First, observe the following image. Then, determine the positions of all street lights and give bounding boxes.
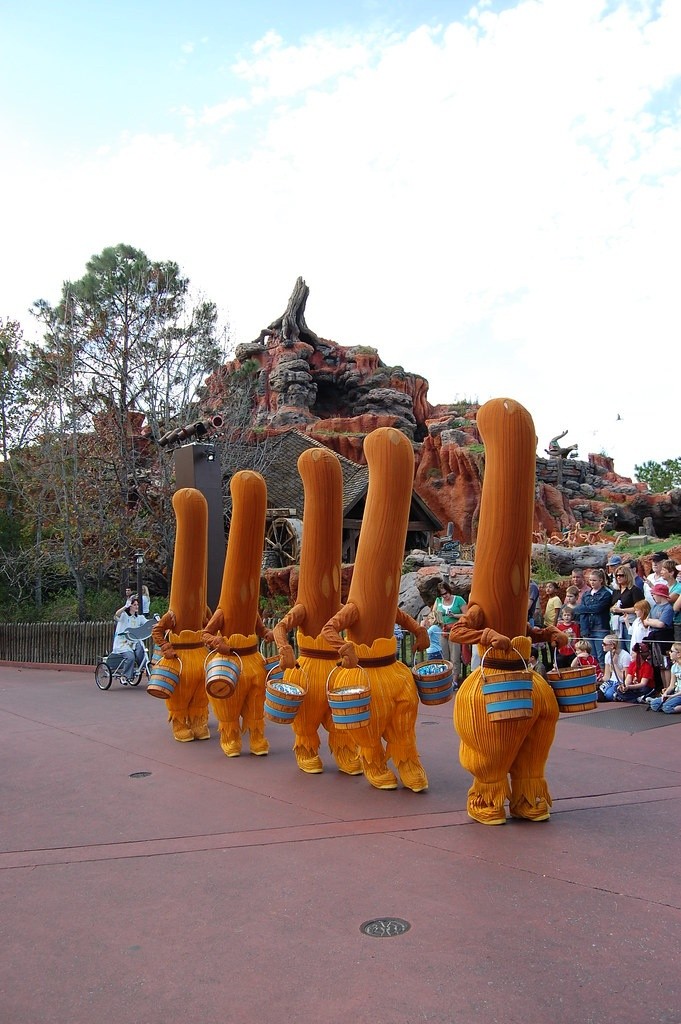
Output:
[134,548,145,615]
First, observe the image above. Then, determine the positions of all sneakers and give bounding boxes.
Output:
[119,676,129,685]
[453,681,459,691]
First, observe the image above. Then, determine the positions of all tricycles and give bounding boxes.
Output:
[95,621,156,690]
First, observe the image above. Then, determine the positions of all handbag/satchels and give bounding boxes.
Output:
[443,622,455,639]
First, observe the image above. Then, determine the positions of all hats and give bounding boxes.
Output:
[632,643,651,656]
[650,584,671,599]
[606,554,622,566]
[649,551,669,562]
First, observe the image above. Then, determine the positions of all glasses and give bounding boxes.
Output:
[439,590,448,596]
[616,573,627,577]
[602,641,612,646]
[670,649,675,653]
[125,589,131,592]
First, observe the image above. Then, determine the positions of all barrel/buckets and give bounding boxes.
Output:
[203,647,244,699]
[411,643,454,705]
[263,663,309,725]
[546,642,597,712]
[260,637,288,680]
[146,655,182,698]
[480,645,533,722]
[324,662,371,730]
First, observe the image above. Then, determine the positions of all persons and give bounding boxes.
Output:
[113,585,150,685]
[420,551,681,714]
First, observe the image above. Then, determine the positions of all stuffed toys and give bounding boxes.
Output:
[449,397,569,826]
[151,488,212,742]
[273,448,363,776]
[203,470,274,757]
[322,427,430,792]
